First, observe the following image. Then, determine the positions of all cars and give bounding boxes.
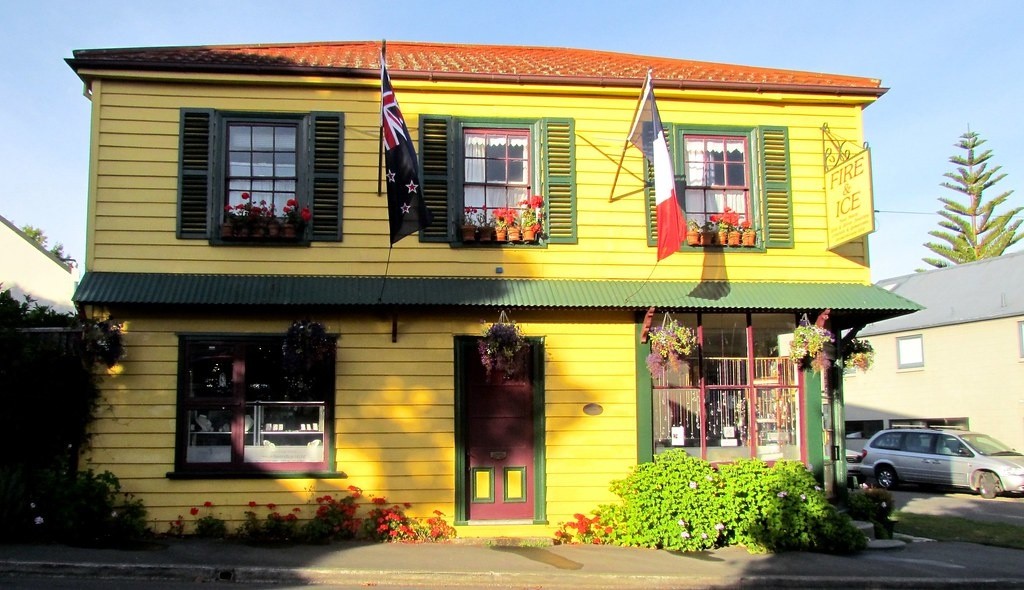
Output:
[845,436,868,486]
[861,428,1024,500]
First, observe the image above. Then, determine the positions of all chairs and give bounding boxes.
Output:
[938,440,951,455]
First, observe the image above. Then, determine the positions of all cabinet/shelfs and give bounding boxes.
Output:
[188,399,325,462]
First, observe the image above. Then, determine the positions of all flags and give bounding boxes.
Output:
[624,66,689,261]
[381,52,433,247]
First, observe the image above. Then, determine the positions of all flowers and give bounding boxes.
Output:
[843,338,874,373]
[646,319,698,379]
[225,193,314,242]
[477,319,552,385]
[688,206,752,232]
[789,326,835,377]
[452,196,547,234]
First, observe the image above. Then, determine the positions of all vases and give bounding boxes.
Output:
[495,227,506,241]
[507,228,520,241]
[700,232,714,246]
[522,227,535,241]
[462,225,477,241]
[686,231,700,246]
[478,226,493,240]
[728,231,740,246]
[716,231,726,245]
[742,231,756,246]
[221,223,296,237]
